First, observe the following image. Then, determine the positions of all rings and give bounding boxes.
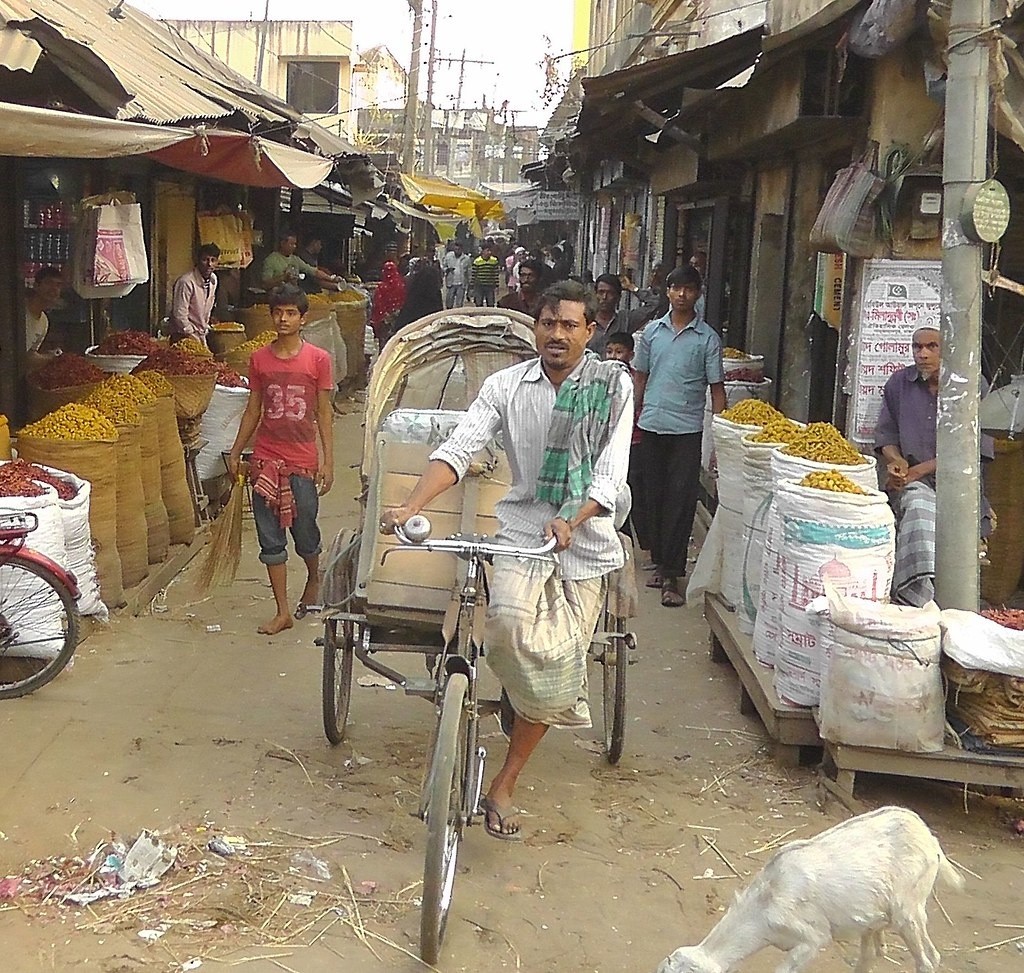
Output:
[379,521,387,529]
[315,483,327,487]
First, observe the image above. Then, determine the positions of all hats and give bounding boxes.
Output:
[514,247,525,255]
[912,315,940,342]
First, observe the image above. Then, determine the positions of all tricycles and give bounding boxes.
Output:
[316,306,639,962]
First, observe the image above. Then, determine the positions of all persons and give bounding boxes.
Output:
[873,327,996,608]
[378,278,633,838]
[228,286,334,636]
[23,268,64,349]
[262,231,726,607]
[170,243,220,347]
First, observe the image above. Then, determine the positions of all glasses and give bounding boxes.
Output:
[670,283,699,292]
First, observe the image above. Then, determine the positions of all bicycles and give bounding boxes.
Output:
[0,514,82,701]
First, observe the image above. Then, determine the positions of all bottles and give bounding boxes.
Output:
[23,263,66,289]
[21,232,71,260]
[19,198,77,229]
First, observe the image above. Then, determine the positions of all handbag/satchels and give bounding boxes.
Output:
[80,197,150,287]
[809,140,885,258]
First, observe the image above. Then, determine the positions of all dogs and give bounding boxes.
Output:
[656,804,967,973]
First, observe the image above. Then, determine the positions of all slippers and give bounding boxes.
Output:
[481,798,522,840]
[500,687,515,735]
[661,584,684,606]
[646,568,663,588]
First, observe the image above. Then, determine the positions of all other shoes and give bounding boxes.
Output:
[639,548,658,570]
